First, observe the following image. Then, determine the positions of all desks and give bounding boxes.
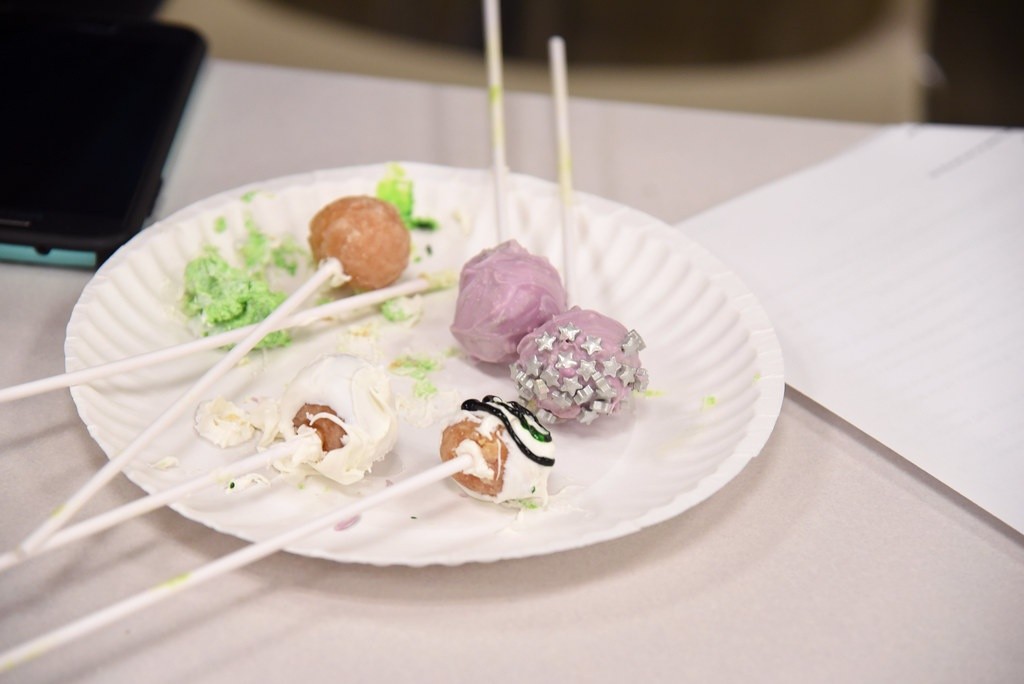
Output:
[0,63,1024,684]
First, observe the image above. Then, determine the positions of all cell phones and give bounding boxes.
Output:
[0,0,208,270]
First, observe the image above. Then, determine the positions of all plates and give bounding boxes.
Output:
[63,159,785,568]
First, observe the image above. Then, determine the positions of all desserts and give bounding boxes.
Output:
[276,193,649,503]
